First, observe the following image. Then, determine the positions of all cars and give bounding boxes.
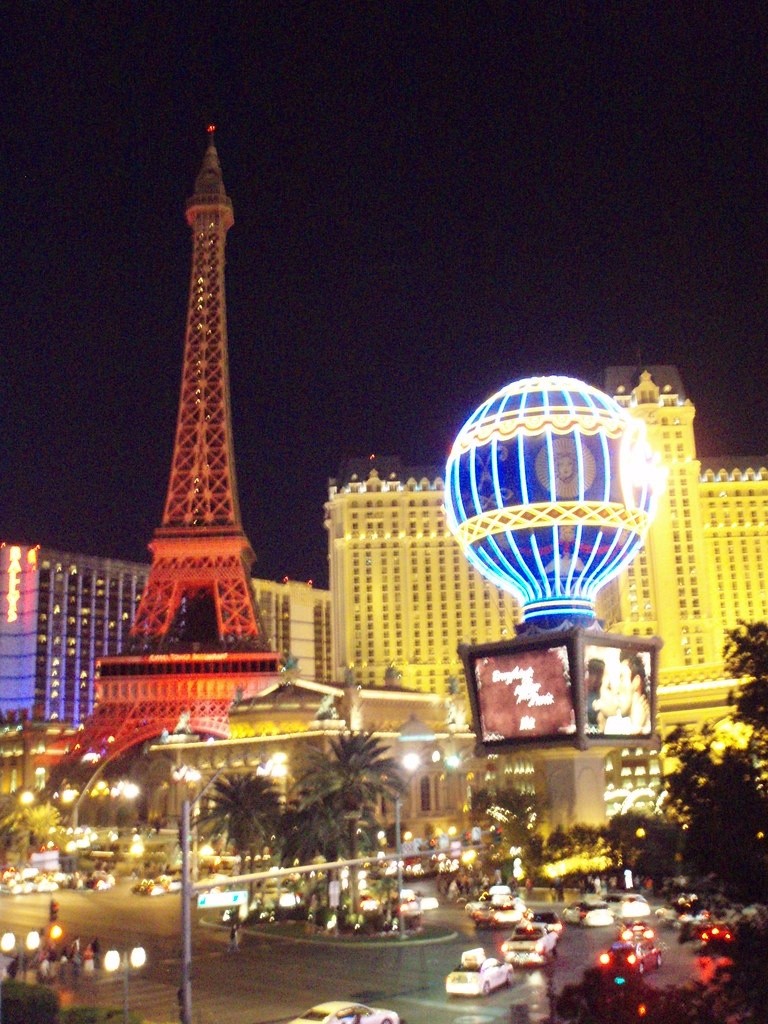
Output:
[462,884,731,969]
[287,998,402,1024]
[445,949,514,996]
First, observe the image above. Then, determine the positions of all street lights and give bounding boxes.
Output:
[179,750,288,1024]
[104,944,147,1023]
[0,929,41,979]
[394,755,461,941]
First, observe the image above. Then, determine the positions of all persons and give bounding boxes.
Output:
[435,866,673,904]
[92,857,264,877]
[585,649,650,734]
[7,937,100,978]
[226,924,239,950]
